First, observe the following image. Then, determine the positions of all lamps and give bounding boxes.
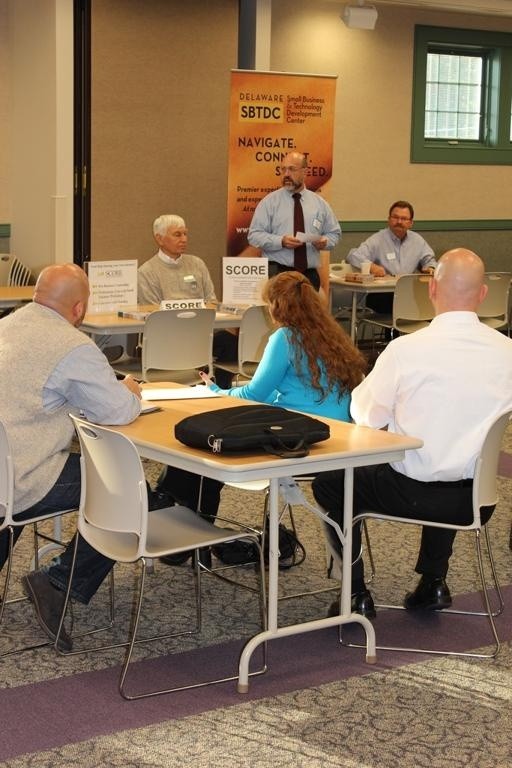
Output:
[338,0,378,30]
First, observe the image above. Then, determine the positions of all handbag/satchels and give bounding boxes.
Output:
[175,405,330,458]
[212,524,296,564]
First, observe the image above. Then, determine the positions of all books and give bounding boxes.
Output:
[140,384,223,401]
[79,400,161,419]
[345,272,396,284]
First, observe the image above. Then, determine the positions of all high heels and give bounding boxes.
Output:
[148,487,175,510]
[159,546,211,570]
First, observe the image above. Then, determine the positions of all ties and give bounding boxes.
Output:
[292,193,307,274]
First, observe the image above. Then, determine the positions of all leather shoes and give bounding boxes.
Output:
[21,566,73,651]
[328,590,376,619]
[405,576,452,612]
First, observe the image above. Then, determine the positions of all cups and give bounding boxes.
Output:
[360,262,371,275]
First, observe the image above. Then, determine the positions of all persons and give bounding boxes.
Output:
[137,214,219,306]
[0,261,142,651]
[158,271,368,571]
[247,151,342,293]
[346,200,437,314]
[311,247,511,620]
